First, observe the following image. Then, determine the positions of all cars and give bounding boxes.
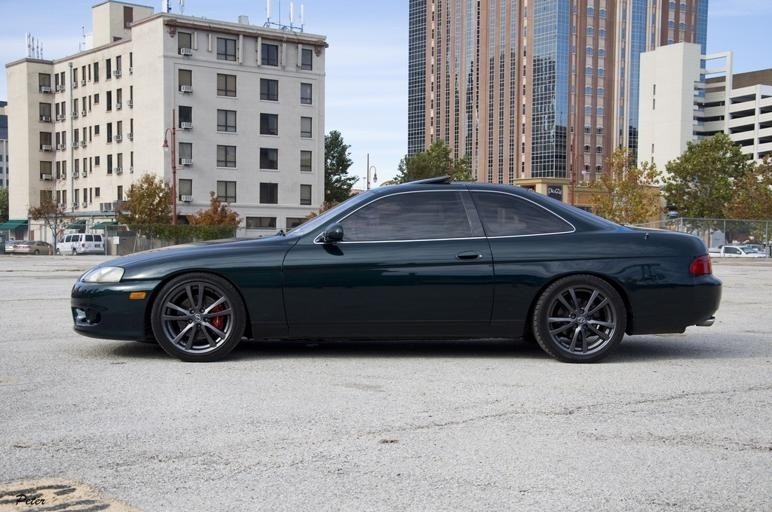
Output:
[70,175,722,363]
[3,240,56,255]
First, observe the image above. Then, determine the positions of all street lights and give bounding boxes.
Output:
[162,127,177,225]
[368,165,378,190]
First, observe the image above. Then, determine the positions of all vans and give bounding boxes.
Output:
[57,233,105,254]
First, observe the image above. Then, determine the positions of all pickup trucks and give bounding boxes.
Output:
[708,243,769,258]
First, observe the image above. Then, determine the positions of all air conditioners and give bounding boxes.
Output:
[181,48,192,56]
[181,195,192,202]
[181,86,193,92]
[181,159,192,164]
[181,121,193,128]
[41,68,133,212]
